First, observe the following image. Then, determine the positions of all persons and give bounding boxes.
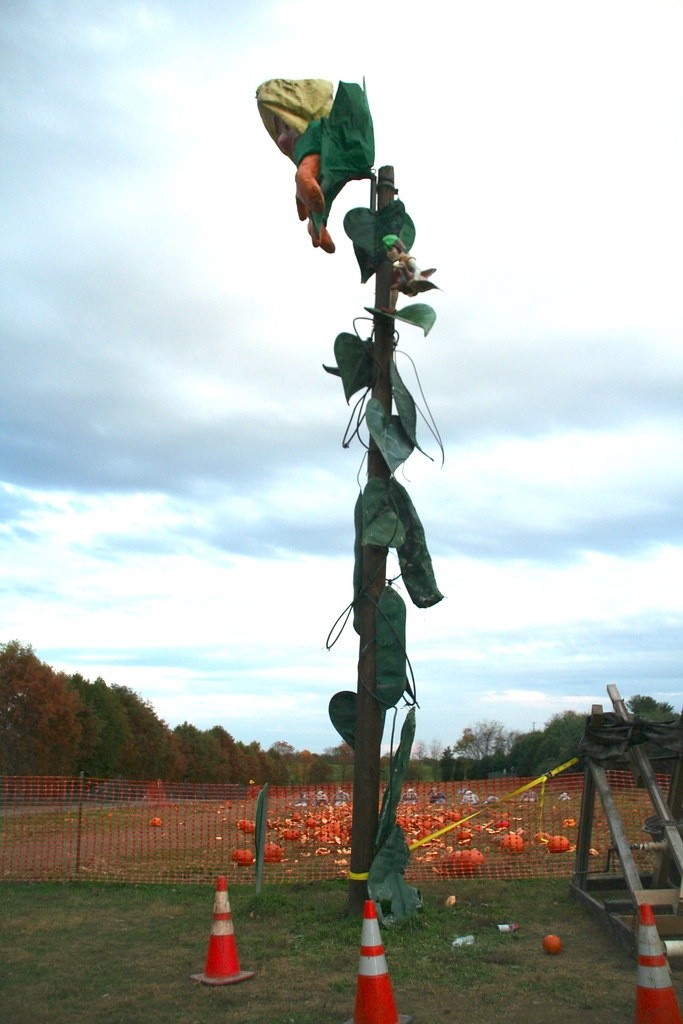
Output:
[400,788,418,805]
[333,789,349,806]
[459,789,479,806]
[312,788,328,806]
[427,785,439,796]
[558,792,571,801]
[520,789,537,802]
[295,793,309,807]
[430,791,445,805]
[483,796,499,805]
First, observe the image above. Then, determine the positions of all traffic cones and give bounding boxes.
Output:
[342,900,414,1024]
[189,875,254,987]
[634,902,683,1023]
